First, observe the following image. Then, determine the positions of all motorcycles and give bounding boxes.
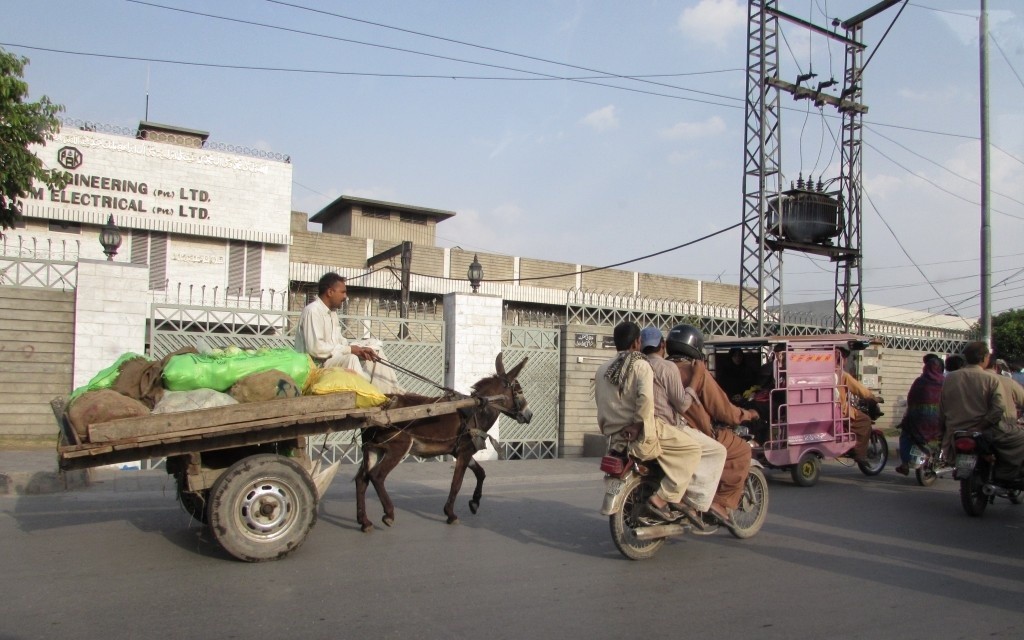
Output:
[950,429,1024,519]
[599,388,769,562]
[703,333,889,489]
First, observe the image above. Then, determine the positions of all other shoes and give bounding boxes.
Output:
[896,464,910,475]
[853,458,871,467]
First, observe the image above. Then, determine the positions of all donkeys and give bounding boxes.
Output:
[354,351,533,532]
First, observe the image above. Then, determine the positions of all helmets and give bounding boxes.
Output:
[666,324,705,360]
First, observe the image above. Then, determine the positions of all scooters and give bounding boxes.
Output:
[906,435,956,488]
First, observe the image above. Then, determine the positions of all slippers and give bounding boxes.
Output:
[646,501,678,521]
[671,502,705,531]
[708,508,738,530]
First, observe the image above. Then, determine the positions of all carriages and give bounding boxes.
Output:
[56,351,534,564]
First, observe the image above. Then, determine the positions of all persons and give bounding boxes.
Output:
[294,272,406,396]
[895,341,1024,489]
[595,322,760,531]
[721,343,786,422]
[835,347,883,463]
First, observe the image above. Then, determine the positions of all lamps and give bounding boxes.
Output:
[98,213,122,262]
[467,253,483,293]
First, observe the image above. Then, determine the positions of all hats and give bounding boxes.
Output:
[639,327,663,352]
